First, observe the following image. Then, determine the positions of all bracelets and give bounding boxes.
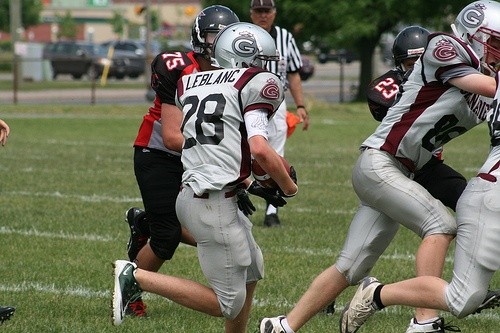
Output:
[297,106,304,108]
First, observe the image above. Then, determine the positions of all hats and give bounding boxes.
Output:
[251,0,275,10]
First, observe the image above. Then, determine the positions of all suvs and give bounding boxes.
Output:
[42,41,162,81]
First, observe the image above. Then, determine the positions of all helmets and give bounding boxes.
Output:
[450,0,500,75]
[392,26,430,66]
[210,21,280,68]
[190,5,240,55]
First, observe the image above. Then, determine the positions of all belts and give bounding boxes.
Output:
[179,185,237,199]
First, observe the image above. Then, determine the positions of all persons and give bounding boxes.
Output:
[111,22,299,333]
[339,70,500,333]
[258,0,500,333]
[247,0,310,227]
[0,119,15,324]
[366,25,500,314]
[124,4,240,316]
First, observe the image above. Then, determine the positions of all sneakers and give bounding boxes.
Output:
[339,276,383,333]
[112,259,146,326]
[406,316,461,333]
[125,206,148,261]
[257,315,290,333]
[264,209,280,226]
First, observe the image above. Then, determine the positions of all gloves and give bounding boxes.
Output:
[247,179,288,208]
[238,188,257,216]
[277,166,300,198]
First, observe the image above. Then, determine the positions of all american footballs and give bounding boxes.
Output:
[251,154,291,189]
